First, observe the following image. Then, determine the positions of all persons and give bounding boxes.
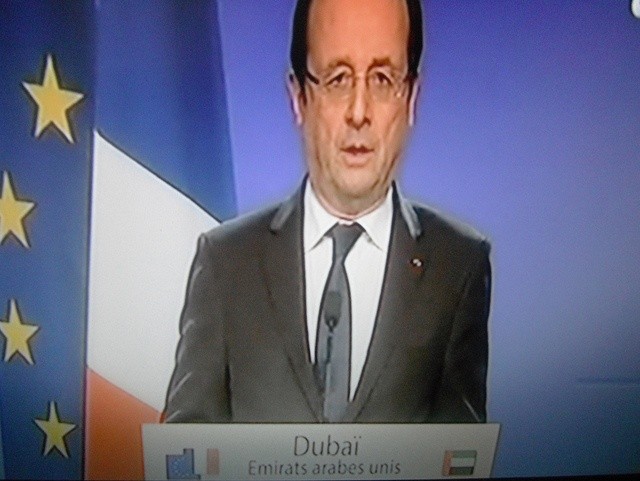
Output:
[159,0,493,424]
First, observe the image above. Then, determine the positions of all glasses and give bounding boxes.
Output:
[299,64,410,103]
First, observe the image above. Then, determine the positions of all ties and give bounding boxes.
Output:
[313,222,366,421]
